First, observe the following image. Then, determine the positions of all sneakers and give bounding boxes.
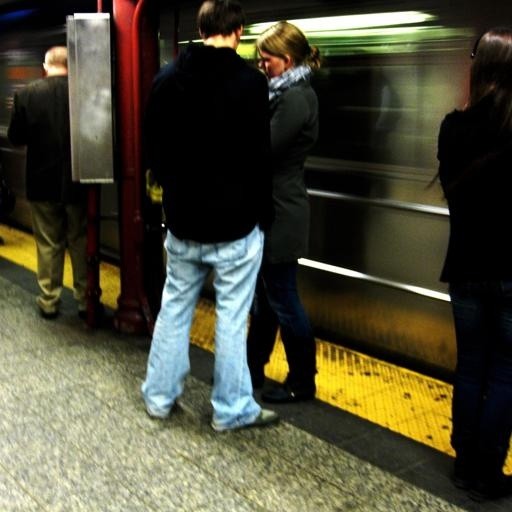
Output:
[39,305,59,320]
[261,380,294,404]
[140,381,169,420]
[211,409,278,432]
[78,302,104,322]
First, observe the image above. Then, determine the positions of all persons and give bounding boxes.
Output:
[251,21,321,405]
[6,45,109,322]
[137,0,284,432]
[424,25,512,505]
